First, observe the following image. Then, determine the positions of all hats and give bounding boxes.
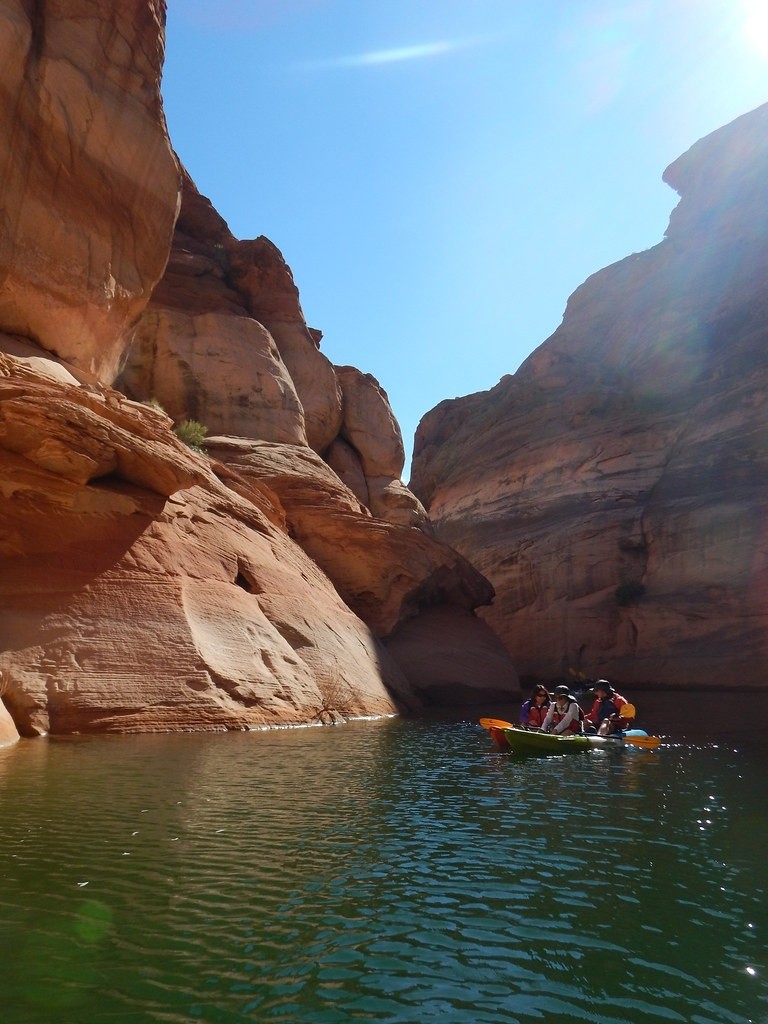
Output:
[548,685,577,702]
[588,680,615,692]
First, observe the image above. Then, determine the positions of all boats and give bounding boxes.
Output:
[502,727,661,757]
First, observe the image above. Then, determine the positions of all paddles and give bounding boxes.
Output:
[615,703,636,719]
[478,715,661,748]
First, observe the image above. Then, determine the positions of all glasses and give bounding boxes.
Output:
[555,696,565,700]
[537,694,547,697]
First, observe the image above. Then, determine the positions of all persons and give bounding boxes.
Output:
[520,684,558,728]
[583,679,630,735]
[538,685,584,736]
